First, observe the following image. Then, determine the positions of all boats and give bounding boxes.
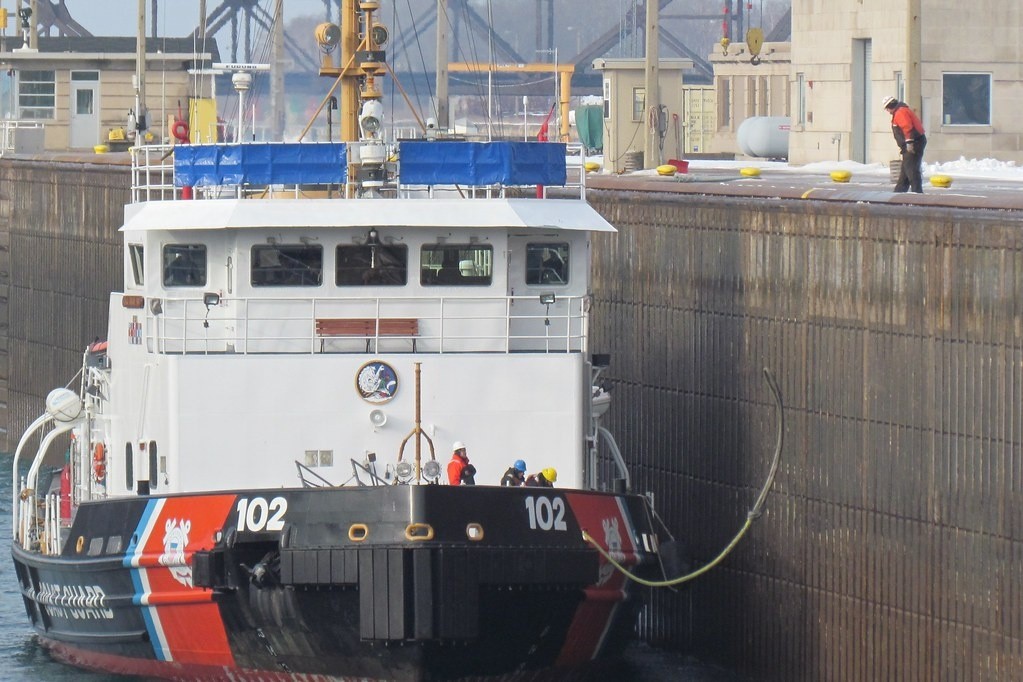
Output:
[7,1,679,682]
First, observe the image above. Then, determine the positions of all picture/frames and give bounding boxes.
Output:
[354,359,400,405]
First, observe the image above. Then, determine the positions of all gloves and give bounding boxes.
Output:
[905,142,916,154]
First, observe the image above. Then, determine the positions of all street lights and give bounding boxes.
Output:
[503,30,519,54]
[535,47,561,142]
[522,95,530,142]
[567,24,581,55]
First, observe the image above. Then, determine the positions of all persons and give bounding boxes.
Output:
[525,468,557,487]
[883,96,927,193]
[529,246,566,285]
[447,442,475,485]
[438,257,462,279]
[500,460,526,486]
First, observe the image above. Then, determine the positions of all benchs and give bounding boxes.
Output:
[314,319,420,353]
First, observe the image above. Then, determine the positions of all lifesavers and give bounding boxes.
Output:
[172,121,189,138]
[95,444,104,475]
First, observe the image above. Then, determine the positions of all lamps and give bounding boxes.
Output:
[539,290,556,352]
[392,462,417,484]
[202,293,220,352]
[420,460,442,484]
[357,100,385,165]
[314,22,341,67]
[370,22,391,50]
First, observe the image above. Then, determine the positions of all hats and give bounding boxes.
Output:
[881,96,895,110]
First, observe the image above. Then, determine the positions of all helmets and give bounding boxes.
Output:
[514,460,527,471]
[541,467,557,482]
[453,442,466,450]
